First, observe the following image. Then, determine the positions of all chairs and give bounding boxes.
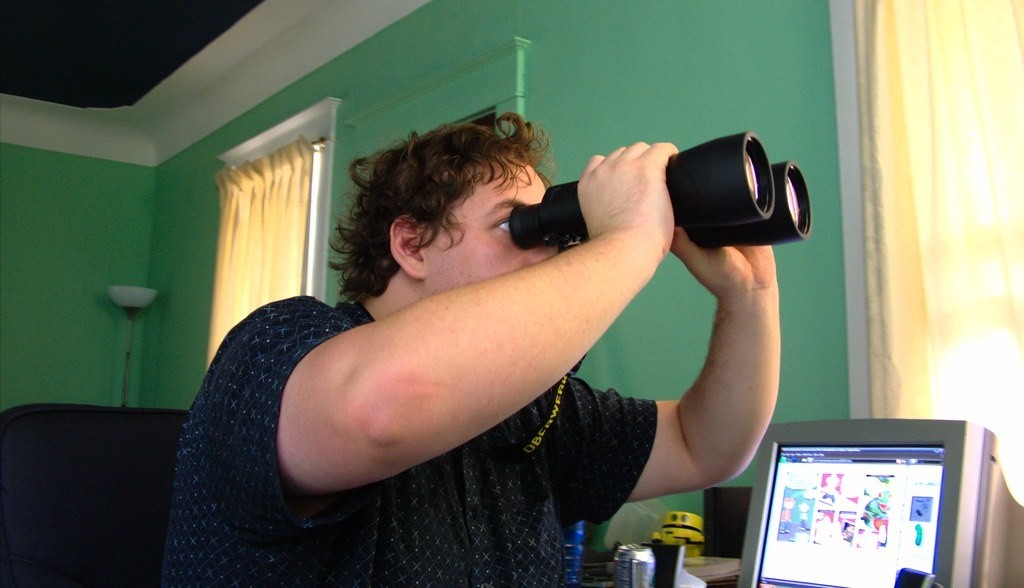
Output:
[0,402,191,588]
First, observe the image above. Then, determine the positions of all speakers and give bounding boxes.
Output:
[703,486,752,559]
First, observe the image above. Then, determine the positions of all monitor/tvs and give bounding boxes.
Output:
[735,419,1024,588]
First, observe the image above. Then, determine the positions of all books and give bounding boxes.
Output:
[683,557,741,582]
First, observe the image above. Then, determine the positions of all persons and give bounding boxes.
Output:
[160,111,782,588]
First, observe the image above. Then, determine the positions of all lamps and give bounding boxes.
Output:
[107,285,160,407]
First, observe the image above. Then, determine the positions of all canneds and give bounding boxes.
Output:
[613,543,657,588]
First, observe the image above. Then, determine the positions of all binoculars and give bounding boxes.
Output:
[508,129,814,249]
